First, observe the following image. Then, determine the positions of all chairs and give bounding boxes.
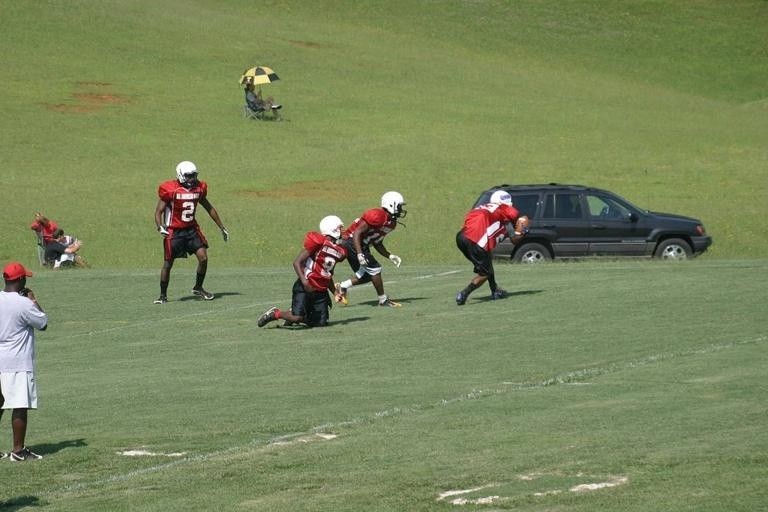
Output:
[242,87,283,121]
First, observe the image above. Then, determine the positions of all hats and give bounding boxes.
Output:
[2,261,33,280]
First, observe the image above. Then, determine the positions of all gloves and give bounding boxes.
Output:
[356,252,370,267]
[222,228,229,242]
[156,224,172,238]
[521,223,530,236]
[387,254,402,267]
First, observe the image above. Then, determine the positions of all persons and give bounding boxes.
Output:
[454,188,530,307]
[246,83,280,120]
[30,212,91,270]
[334,189,407,308]
[257,214,350,328]
[0,260,50,462]
[153,158,229,306]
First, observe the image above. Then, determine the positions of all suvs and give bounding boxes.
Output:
[471,180,713,264]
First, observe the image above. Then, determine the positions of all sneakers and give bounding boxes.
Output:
[192,287,215,300]
[0,445,43,462]
[333,281,348,306]
[456,289,468,306]
[153,295,168,304]
[256,306,280,327]
[377,294,402,308]
[490,288,510,300]
[271,104,282,109]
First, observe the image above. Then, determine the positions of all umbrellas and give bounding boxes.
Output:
[238,64,281,119]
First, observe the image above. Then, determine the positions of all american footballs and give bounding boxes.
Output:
[515,216,528,236]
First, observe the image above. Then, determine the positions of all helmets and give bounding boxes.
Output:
[176,160,199,187]
[489,190,513,207]
[319,215,344,240]
[381,191,405,215]
